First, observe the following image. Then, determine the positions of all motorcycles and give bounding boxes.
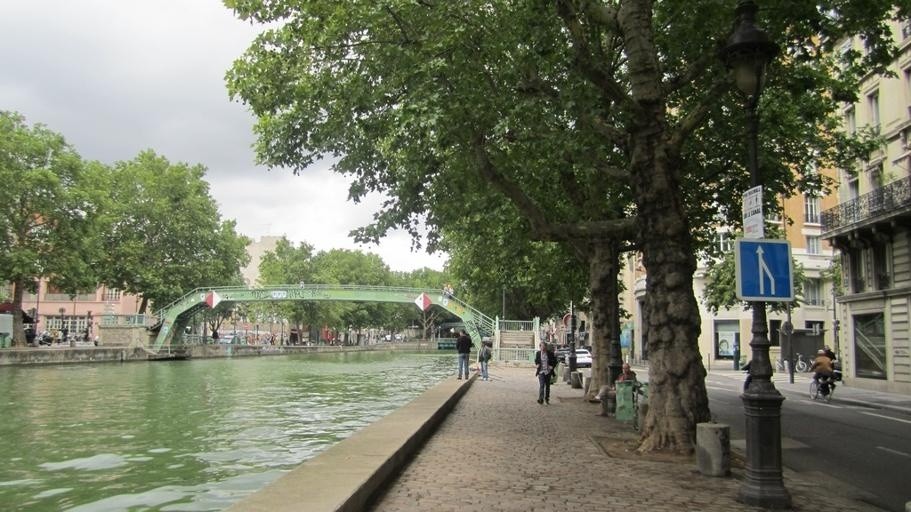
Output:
[776,352,816,373]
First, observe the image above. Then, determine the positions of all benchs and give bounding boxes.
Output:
[602,372,641,414]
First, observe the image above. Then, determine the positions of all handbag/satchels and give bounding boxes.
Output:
[479,354,483,362]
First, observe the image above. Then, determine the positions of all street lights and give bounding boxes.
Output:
[244,325,249,344]
[717,1,798,512]
[438,326,442,342]
[257,322,260,350]
[349,326,353,345]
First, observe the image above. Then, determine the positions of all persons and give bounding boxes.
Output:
[448,285,454,298]
[742,359,752,391]
[443,284,448,296]
[456,329,471,379]
[535,342,556,404]
[825,345,836,370]
[809,349,832,392]
[590,363,636,416]
[478,342,492,380]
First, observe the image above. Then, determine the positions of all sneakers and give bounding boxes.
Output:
[588,398,608,417]
[457,377,469,380]
[536,398,551,406]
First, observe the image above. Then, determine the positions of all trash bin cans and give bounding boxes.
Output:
[32,338,39,347]
[770,346,782,371]
[614,380,638,421]
[0,333,12,348]
[240,336,246,344]
[70,340,76,347]
[357,334,365,345]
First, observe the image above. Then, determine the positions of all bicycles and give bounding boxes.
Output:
[808,360,836,402]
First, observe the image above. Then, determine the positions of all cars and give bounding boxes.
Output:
[24,328,94,346]
[219,334,241,344]
[381,332,404,342]
[302,331,310,343]
[549,343,593,366]
